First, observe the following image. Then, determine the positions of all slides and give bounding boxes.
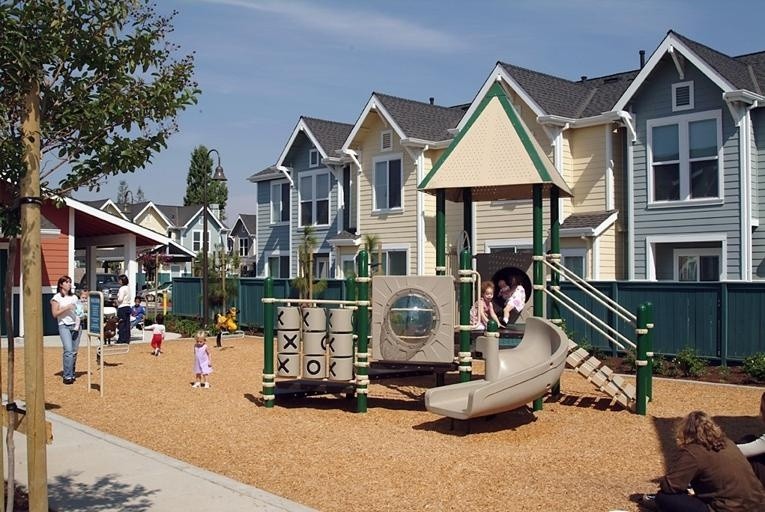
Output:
[425,317,568,420]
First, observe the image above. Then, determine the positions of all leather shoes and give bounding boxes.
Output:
[63,377,72,384]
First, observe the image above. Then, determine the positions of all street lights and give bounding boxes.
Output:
[120,189,134,224]
[203,146,228,332]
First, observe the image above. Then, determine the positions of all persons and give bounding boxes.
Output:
[734,392,765,487]
[192,331,213,388]
[469,281,507,328]
[50,276,82,384]
[112,275,131,344]
[130,296,146,330]
[71,289,88,332]
[499,275,526,326]
[144,314,166,356]
[656,411,765,512]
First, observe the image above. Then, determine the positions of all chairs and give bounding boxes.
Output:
[129,298,148,341]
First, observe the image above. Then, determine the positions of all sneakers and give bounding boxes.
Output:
[204,382,209,389]
[192,382,200,388]
[155,348,160,356]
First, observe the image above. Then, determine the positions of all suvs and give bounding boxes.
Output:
[81,273,123,306]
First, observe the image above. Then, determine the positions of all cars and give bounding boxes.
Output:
[135,281,172,303]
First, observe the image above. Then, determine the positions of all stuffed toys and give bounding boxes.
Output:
[496,280,511,300]
